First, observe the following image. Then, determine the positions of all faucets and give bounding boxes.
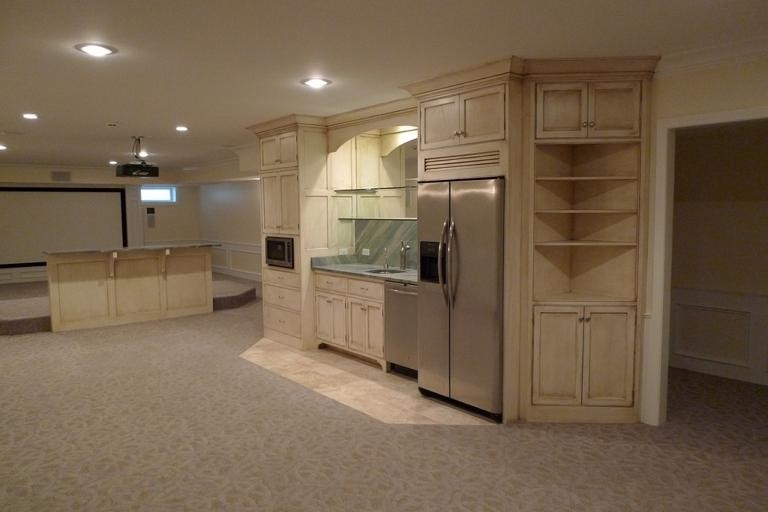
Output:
[400,241,407,270]
[405,244,411,249]
[384,247,388,267]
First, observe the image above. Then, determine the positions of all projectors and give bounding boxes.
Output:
[115,164,159,177]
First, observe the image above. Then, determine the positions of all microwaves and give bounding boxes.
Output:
[265,236,294,269]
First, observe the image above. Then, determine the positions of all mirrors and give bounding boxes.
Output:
[356,188,418,219]
[379,128,418,187]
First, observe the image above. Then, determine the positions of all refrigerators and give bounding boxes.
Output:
[417,177,506,418]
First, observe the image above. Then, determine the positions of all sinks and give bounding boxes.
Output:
[365,268,404,274]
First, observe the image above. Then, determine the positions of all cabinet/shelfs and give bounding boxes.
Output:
[535,80,642,138]
[534,141,638,303]
[260,171,328,235]
[315,272,347,347]
[420,83,506,150]
[263,267,313,339]
[328,126,381,191]
[349,278,384,360]
[532,305,636,407]
[259,128,328,170]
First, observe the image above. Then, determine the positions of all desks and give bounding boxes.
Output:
[41,240,222,333]
